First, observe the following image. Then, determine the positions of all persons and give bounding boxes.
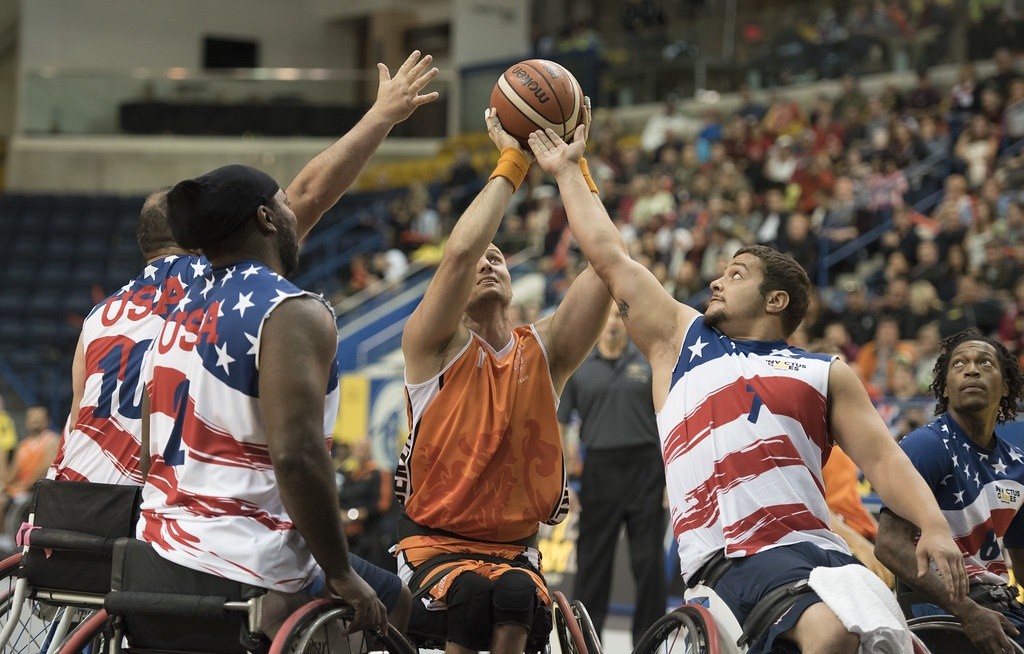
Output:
[530,125,968,654]
[0,0,1024,591]
[387,109,631,654]
[874,327,1024,654]
[134,163,412,637]
[46,48,439,486]
[555,301,665,653]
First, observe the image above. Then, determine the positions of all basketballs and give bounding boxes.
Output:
[488,59,585,156]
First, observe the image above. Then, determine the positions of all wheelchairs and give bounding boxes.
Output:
[630,568,1024,654]
[0,476,604,654]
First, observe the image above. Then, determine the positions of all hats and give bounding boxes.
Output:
[166,164,279,250]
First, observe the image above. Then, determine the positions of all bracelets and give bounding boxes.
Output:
[488,147,530,191]
[580,158,599,194]
